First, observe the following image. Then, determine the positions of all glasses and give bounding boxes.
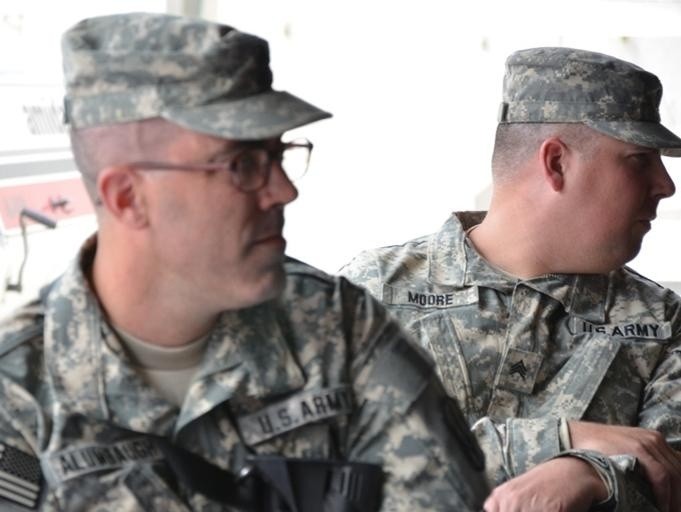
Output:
[126,135,313,194]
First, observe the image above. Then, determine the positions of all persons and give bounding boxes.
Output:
[1,12,487,511]
[331,46,681,509]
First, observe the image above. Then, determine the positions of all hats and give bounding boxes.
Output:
[59,10,333,144]
[498,46,681,158]
